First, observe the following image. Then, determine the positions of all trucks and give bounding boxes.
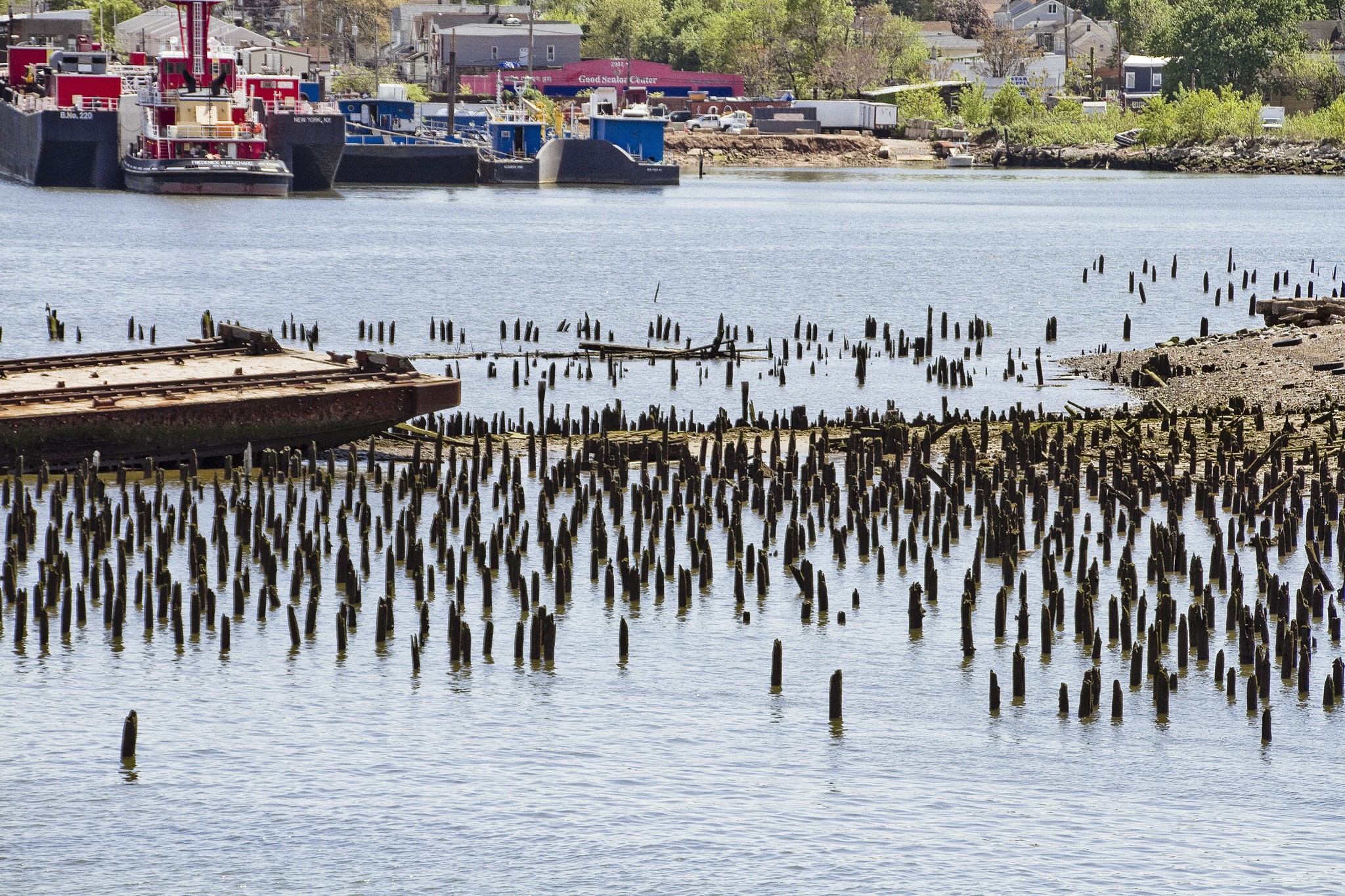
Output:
[1259,107,1285,131]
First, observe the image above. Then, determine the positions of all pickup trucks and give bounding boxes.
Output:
[684,114,748,132]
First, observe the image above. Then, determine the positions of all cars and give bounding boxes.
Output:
[727,123,746,133]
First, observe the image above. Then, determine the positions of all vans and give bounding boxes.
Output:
[664,110,693,122]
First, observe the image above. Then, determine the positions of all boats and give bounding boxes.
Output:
[1113,127,1151,148]
[120,0,295,198]
